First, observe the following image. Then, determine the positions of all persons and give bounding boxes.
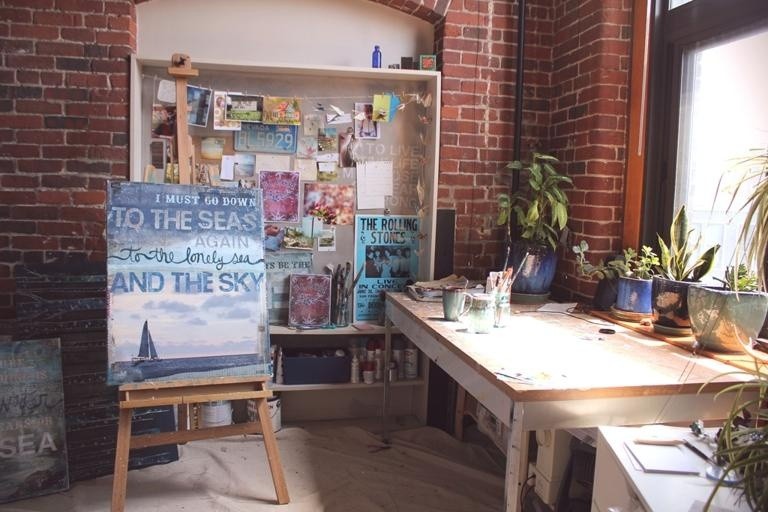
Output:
[360,105,378,138]
[367,246,410,277]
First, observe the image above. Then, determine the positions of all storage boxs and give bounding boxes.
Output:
[280,343,353,385]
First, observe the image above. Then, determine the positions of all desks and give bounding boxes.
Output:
[378,284,768,512]
[589,423,768,511]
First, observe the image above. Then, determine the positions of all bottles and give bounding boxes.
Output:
[371,44,383,68]
[347,336,418,385]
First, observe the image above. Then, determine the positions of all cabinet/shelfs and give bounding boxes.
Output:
[128,54,444,441]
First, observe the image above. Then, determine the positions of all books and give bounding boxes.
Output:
[623,438,701,474]
[405,274,488,303]
[287,273,333,328]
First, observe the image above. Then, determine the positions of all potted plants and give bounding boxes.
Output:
[686,263,768,354]
[573,237,621,310]
[495,154,571,293]
[610,244,658,313]
[649,205,720,336]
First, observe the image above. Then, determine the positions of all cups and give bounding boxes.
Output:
[472,292,494,334]
[486,292,511,328]
[442,286,474,322]
